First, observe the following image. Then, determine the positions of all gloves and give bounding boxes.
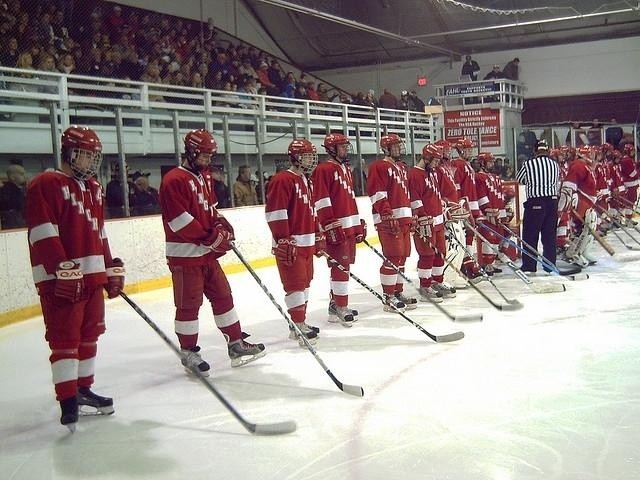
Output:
[314,233,327,258]
[104,258,124,299]
[382,216,400,235]
[276,239,297,267]
[410,215,433,239]
[444,201,513,230]
[201,218,235,254]
[355,220,367,244]
[53,261,84,303]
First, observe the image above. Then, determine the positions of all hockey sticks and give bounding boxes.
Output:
[467,220,589,293]
[230,242,365,395]
[321,220,522,343]
[118,290,296,434]
[571,193,640,262]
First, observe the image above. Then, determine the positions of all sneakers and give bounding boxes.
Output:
[460,263,502,280]
[557,216,638,252]
[288,321,320,339]
[520,265,536,272]
[419,282,456,299]
[60,386,112,424]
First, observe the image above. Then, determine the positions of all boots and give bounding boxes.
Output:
[328,290,359,321]
[180,346,210,370]
[383,289,417,307]
[224,333,265,358]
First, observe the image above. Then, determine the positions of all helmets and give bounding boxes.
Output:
[288,141,318,174]
[477,152,496,162]
[324,133,353,162]
[185,129,217,171]
[381,135,406,163]
[60,127,103,182]
[423,138,473,172]
[536,140,634,166]
